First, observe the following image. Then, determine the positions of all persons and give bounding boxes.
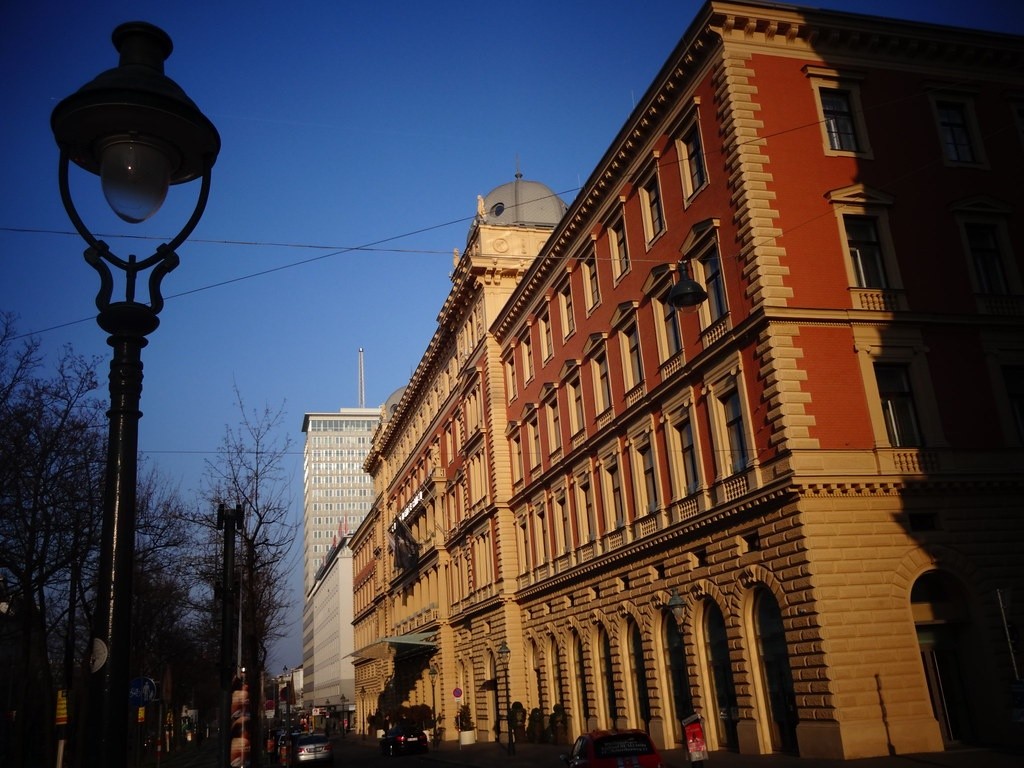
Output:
[181,719,189,743]
[143,682,151,701]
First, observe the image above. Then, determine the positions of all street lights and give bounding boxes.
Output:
[428,665,439,751]
[46,16,224,768]
[498,640,517,755]
[358,686,367,740]
[340,694,346,738]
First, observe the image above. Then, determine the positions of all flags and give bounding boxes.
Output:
[394,515,420,569]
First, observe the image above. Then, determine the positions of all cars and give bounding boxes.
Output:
[266,718,336,768]
[560,728,668,768]
[379,724,429,757]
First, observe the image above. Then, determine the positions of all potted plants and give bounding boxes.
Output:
[455,702,476,744]
